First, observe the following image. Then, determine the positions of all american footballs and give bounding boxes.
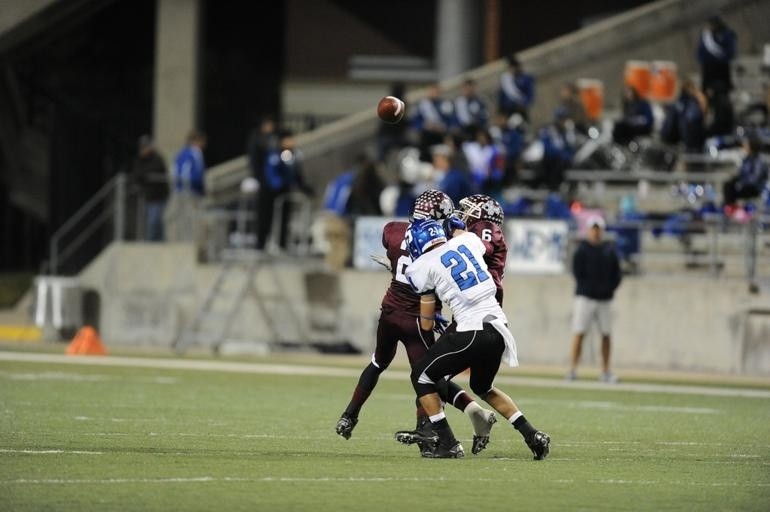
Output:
[379,96,405,124]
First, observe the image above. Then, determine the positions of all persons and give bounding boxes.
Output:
[333,188,454,442]
[402,216,550,461]
[253,128,318,250]
[246,115,277,179]
[563,214,624,384]
[136,134,170,239]
[168,130,209,246]
[320,13,770,280]
[395,192,507,454]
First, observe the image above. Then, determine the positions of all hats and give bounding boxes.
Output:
[587,215,607,230]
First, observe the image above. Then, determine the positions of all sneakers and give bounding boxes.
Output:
[600,372,619,382]
[532,431,550,459]
[335,412,358,439]
[472,410,496,455]
[394,422,438,445]
[567,369,576,380]
[422,441,464,458]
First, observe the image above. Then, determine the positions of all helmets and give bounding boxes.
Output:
[405,218,447,261]
[413,189,455,221]
[459,194,504,223]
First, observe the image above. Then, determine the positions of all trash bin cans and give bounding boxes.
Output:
[35,276,101,341]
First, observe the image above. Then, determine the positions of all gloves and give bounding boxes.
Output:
[370,251,391,270]
[443,217,465,240]
[432,314,450,334]
